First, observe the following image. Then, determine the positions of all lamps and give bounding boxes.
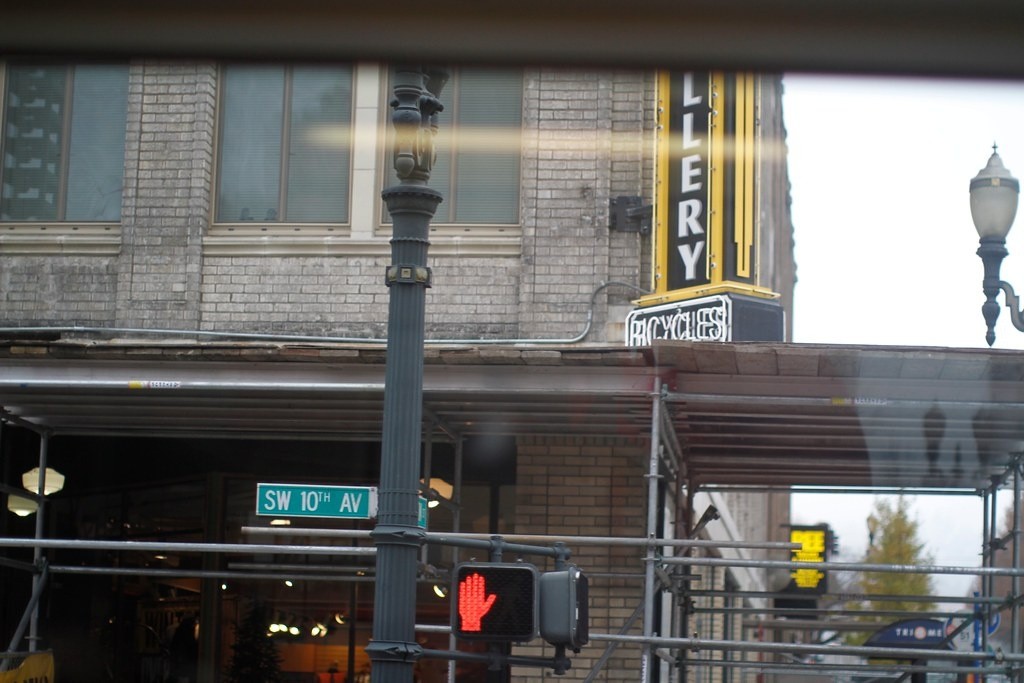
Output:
[432,583,449,599]
[968,141,1024,346]
[419,478,453,508]
[303,623,320,637]
[289,616,304,636]
[278,613,294,633]
[269,610,284,633]
[22,467,65,496]
[7,495,38,517]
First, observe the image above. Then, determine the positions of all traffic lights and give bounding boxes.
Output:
[452,563,539,644]
[538,568,590,653]
